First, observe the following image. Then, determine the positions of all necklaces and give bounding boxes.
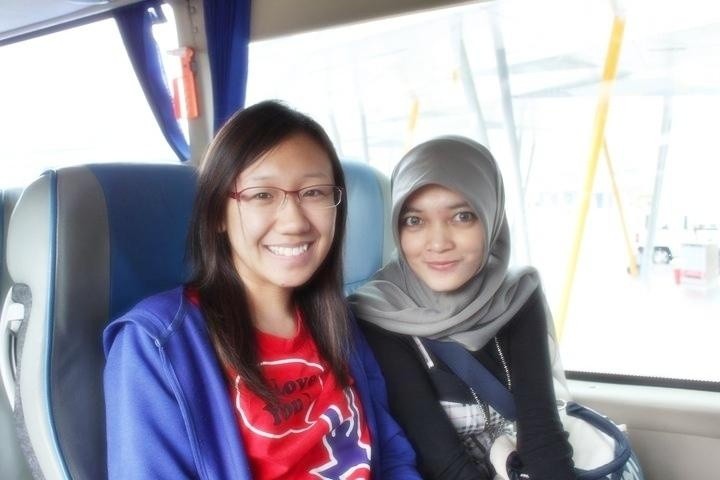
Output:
[470,335,511,441]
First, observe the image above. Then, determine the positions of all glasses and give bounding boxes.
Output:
[229,183,345,215]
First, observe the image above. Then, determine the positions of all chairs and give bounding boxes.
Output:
[2,159,220,480]
[332,153,402,305]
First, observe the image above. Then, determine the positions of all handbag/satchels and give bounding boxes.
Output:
[491,401,647,480]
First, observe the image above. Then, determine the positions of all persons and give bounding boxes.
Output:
[101,99,423,480]
[346,136,643,480]
